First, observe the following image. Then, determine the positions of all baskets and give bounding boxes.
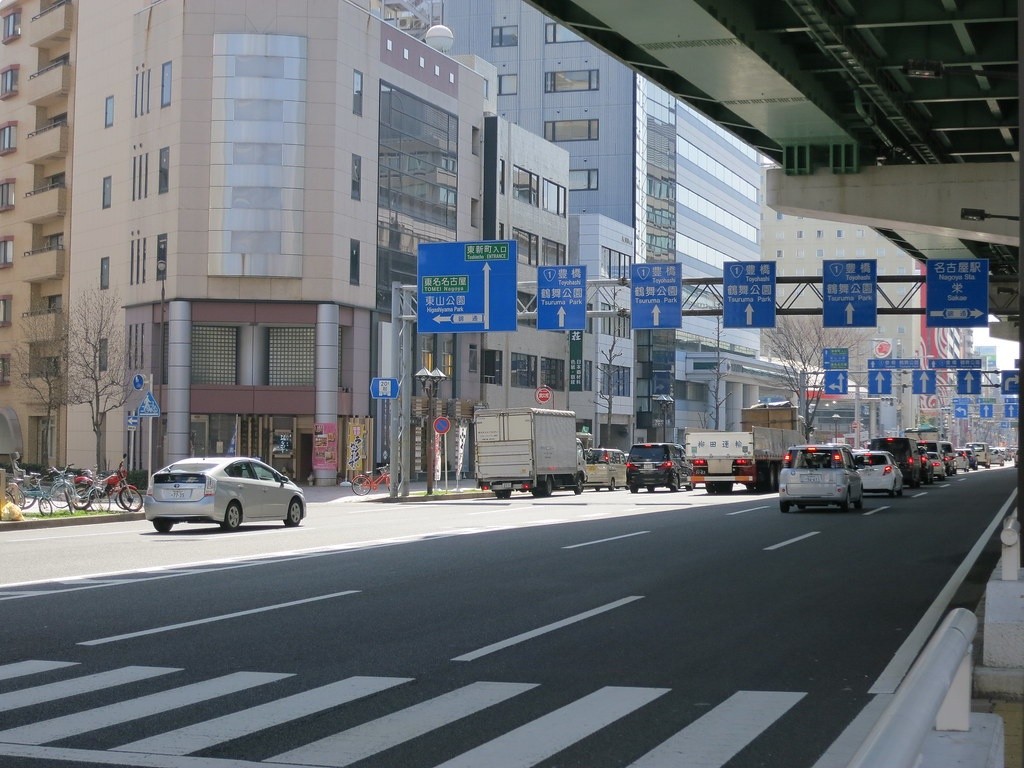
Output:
[95,474,108,487]
[53,472,74,486]
[380,467,390,475]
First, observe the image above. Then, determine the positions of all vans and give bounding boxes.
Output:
[918,440,957,475]
[966,443,991,468]
[869,438,924,489]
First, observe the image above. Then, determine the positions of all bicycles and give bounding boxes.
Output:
[351,464,392,496]
[7,462,132,513]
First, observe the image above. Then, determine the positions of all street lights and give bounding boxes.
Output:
[657,395,675,443]
[412,366,447,495]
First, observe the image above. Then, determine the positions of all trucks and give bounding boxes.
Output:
[686,406,814,495]
[473,406,587,500]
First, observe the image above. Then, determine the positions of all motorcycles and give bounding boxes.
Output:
[72,452,143,512]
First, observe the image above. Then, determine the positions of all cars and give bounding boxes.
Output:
[584,437,1019,513]
[145,454,307,532]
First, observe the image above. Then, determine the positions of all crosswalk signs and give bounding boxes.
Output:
[139,390,160,417]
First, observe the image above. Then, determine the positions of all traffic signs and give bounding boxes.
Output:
[723,261,776,329]
[868,359,983,369]
[631,262,682,330]
[823,348,849,369]
[537,266,586,331]
[417,239,518,333]
[823,259,877,328]
[926,259,988,327]
